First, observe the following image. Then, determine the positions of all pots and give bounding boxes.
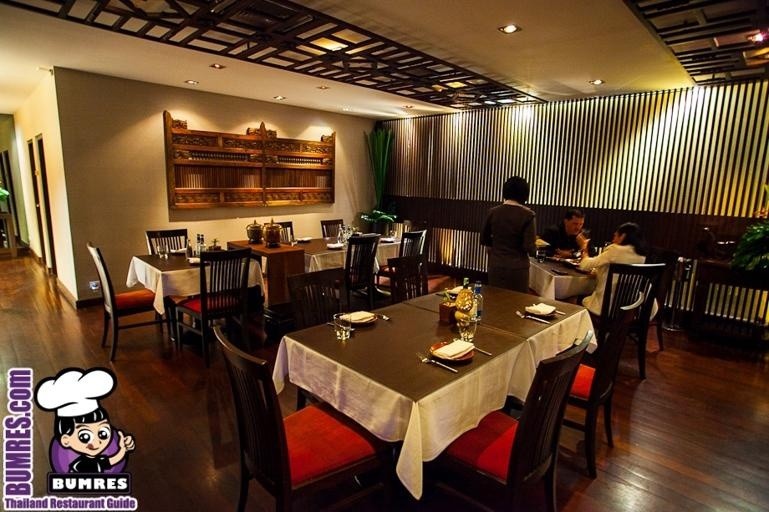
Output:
[246,219,263,239]
[262,219,285,243]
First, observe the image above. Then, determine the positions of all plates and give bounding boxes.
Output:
[170,251,186,256]
[188,258,209,265]
[328,245,344,249]
[430,342,474,362]
[296,237,312,242]
[340,314,377,324]
[524,306,558,317]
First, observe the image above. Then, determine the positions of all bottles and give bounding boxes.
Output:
[200,235,206,252]
[463,277,469,288]
[472,280,483,324]
[185,239,192,259]
[195,234,200,256]
[443,288,450,302]
[337,223,359,243]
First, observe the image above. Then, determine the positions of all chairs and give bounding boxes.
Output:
[691,227,738,261]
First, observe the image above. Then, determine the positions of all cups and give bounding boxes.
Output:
[536,250,546,263]
[333,312,350,340]
[458,318,477,341]
[157,246,169,260]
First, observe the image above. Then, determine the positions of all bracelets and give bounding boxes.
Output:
[580,248,589,255]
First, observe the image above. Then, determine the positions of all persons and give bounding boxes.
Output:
[568,222,648,320]
[480,176,538,294]
[541,206,597,261]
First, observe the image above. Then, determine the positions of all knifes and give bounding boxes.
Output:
[327,322,355,331]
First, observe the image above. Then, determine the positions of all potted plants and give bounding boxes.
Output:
[360,123,396,235]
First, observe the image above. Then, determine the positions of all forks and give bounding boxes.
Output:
[416,351,458,374]
[376,313,392,320]
[515,310,551,324]
